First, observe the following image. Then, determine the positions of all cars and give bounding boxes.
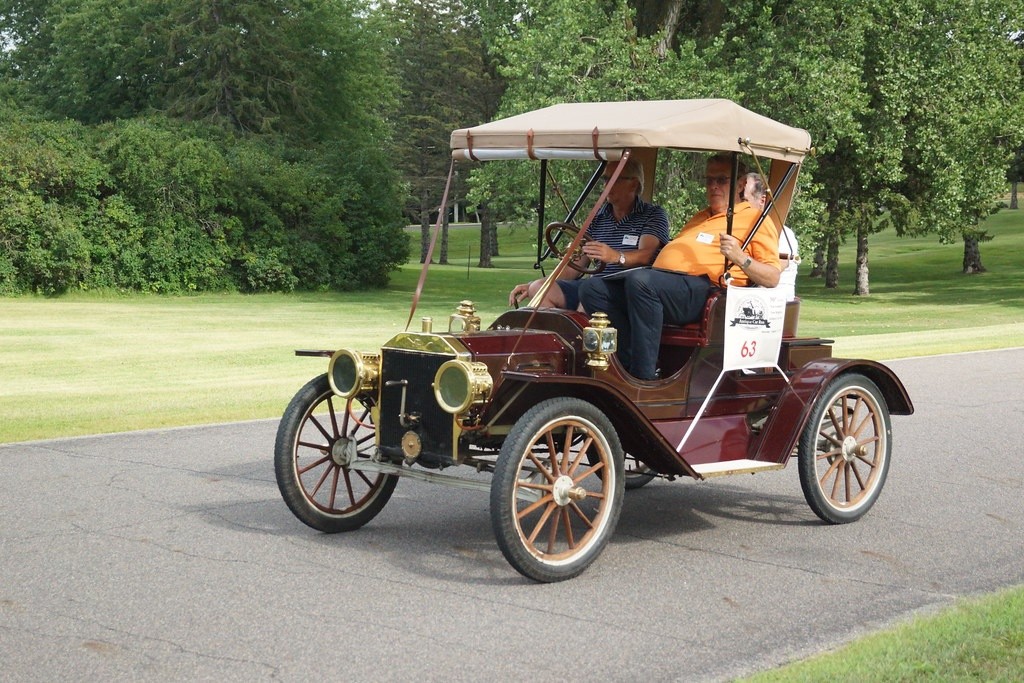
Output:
[274,97,914,585]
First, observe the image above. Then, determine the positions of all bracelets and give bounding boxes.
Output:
[738,256,753,271]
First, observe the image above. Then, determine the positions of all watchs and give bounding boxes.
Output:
[578,154,782,381]
[617,251,626,266]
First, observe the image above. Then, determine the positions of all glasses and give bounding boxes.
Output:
[600,175,634,183]
[704,177,730,185]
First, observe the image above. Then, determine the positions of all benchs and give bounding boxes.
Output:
[660,283,760,345]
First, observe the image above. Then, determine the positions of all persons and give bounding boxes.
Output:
[736,170,799,303]
[507,157,671,365]
